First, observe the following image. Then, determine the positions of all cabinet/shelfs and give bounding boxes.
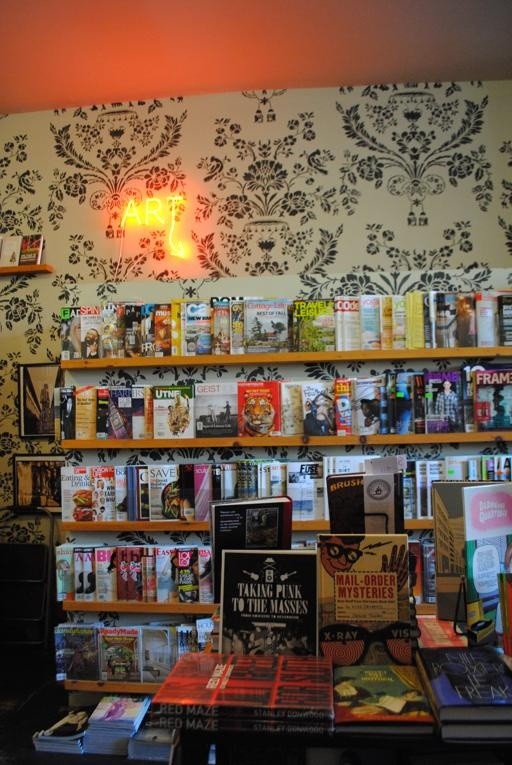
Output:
[55,348,512,696]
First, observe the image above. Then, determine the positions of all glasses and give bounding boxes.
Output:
[321,621,420,666]
[321,541,362,563]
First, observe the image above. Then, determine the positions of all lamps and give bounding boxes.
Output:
[100,194,192,325]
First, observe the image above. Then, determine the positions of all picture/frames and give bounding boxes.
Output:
[12,453,66,515]
[18,362,56,436]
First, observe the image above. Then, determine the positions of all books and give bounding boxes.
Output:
[0,236,23,267]
[19,234,43,266]
[31,290,512,760]
